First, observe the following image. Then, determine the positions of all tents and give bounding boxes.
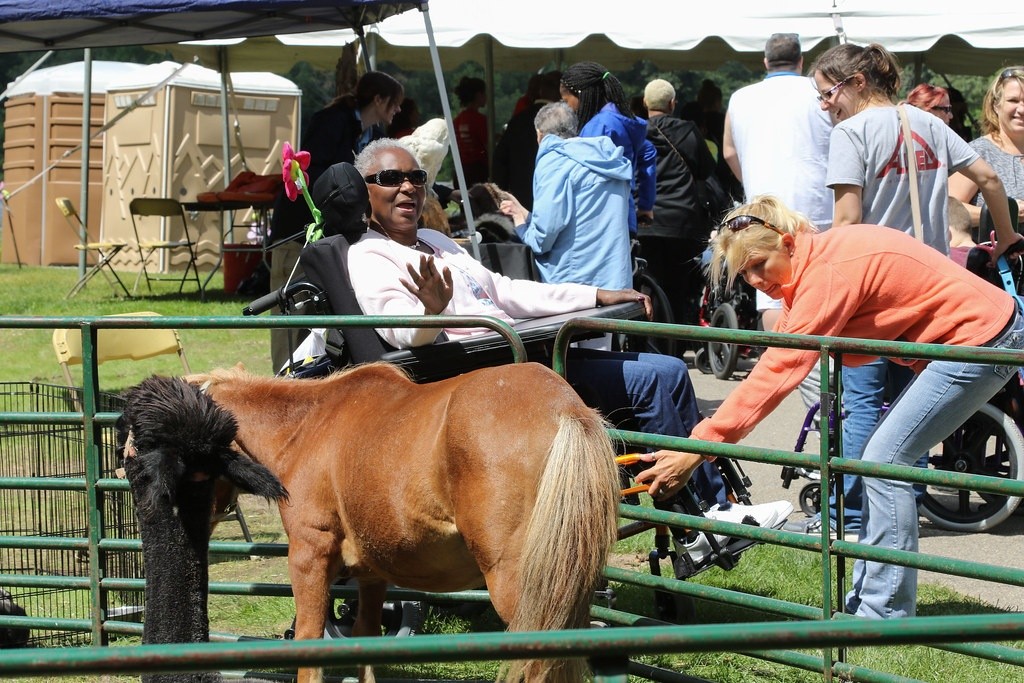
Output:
[0,0,484,277]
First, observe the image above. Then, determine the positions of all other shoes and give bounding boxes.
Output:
[779,517,859,542]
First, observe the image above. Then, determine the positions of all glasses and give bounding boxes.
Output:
[999,68,1024,84]
[715,215,784,236]
[930,105,952,114]
[817,74,857,102]
[363,168,427,187]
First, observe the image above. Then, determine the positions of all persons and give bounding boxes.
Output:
[342,135,789,574]
[447,30,1023,536]
[635,195,1023,623]
[266,68,407,379]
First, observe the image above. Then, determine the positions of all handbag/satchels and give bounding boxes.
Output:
[696,175,735,223]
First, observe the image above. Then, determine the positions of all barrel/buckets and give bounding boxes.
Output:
[221,243,263,295]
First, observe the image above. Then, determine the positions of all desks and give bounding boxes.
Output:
[180,200,274,298]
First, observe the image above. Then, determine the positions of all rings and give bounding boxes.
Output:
[652,451,655,461]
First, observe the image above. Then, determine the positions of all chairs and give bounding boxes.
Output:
[51,310,262,563]
[129,198,202,298]
[54,196,134,300]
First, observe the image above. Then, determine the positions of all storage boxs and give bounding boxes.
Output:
[223,244,270,294]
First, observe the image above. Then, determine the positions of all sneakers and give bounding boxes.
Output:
[677,506,777,568]
[701,500,795,555]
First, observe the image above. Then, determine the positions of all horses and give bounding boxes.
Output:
[119,361,622,683]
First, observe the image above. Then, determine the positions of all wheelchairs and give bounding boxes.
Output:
[630,236,678,357]
[693,267,763,379]
[778,240,1024,533]
[240,162,767,580]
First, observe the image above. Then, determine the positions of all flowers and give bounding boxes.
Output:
[282,141,325,243]
